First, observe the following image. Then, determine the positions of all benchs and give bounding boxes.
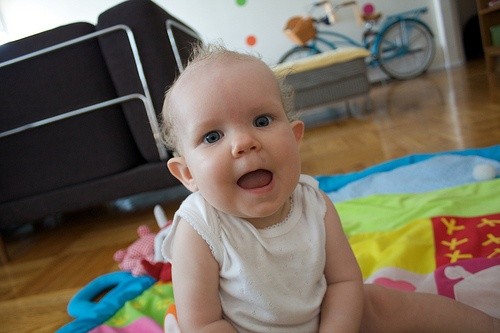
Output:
[271,45,370,116]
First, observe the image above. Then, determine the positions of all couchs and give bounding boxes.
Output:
[0,0,203,235]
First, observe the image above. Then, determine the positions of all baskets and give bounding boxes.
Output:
[284,14,316,45]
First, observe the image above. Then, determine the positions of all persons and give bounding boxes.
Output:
[158,43,497,331]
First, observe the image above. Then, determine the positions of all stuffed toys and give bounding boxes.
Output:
[57,205,173,333]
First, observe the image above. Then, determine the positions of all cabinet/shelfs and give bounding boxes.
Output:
[476,0,500,75]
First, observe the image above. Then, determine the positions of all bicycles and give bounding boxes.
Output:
[277,6,436,81]
